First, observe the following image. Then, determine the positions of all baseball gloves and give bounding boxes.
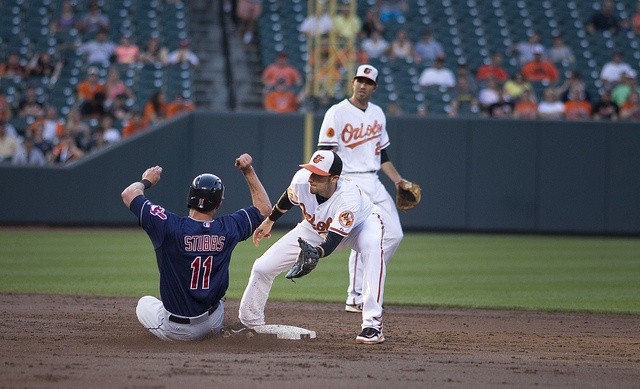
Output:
[285,237,321,278]
[396,181,422,210]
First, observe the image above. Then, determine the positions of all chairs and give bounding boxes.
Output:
[0,1,193,167]
[258,2,639,113]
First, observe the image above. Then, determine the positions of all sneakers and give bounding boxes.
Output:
[213,321,258,340]
[345,302,384,312]
[356,328,386,344]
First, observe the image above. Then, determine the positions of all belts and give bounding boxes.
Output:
[170,301,222,324]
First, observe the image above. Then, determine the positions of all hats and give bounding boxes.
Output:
[299,149,342,173]
[354,64,378,86]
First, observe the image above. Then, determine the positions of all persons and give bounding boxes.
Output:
[120,151,274,344]
[237,0,261,45]
[238,149,388,345]
[303,1,456,92]
[317,64,408,314]
[262,51,301,87]
[0,0,198,168]
[585,2,618,35]
[261,75,304,115]
[451,31,640,121]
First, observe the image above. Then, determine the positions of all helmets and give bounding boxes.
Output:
[187,172,225,211]
